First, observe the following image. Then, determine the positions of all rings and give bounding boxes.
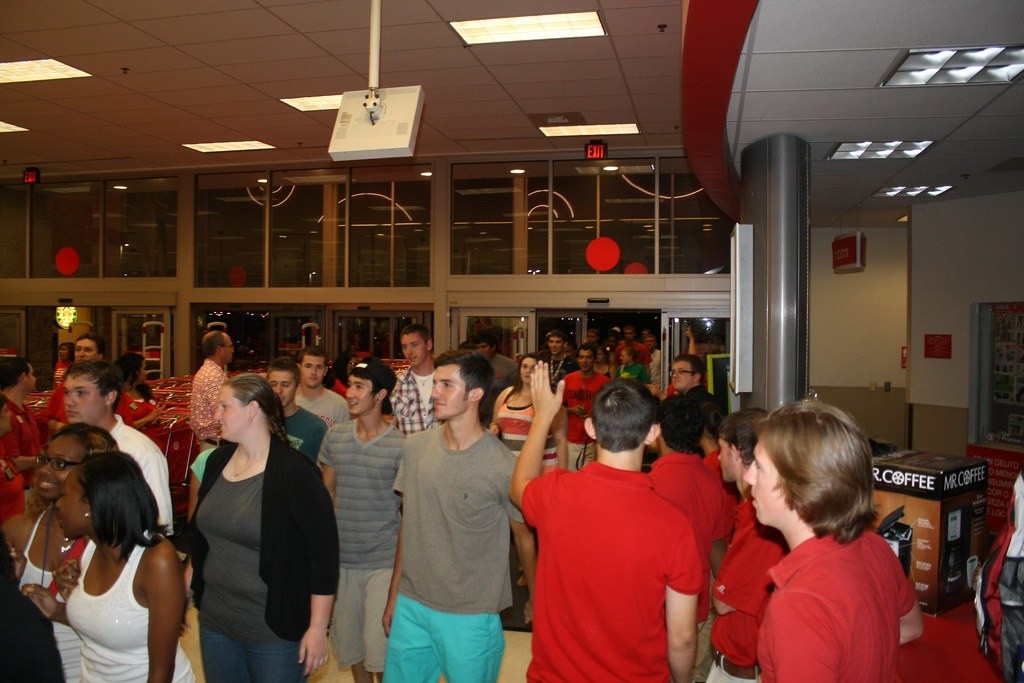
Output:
[28,581,35,588]
[68,573,73,581]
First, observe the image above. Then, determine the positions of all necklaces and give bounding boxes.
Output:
[232,450,250,475]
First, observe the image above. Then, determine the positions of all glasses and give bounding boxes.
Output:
[37,455,80,471]
[669,367,695,378]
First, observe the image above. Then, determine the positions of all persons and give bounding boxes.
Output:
[0,323,928,683]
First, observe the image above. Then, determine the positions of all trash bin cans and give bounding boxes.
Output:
[870,450,988,618]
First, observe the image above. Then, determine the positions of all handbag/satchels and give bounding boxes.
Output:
[24,483,35,490]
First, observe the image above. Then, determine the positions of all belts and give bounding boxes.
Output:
[714,650,760,680]
[205,438,217,446]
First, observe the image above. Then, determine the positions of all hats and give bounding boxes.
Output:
[354,356,396,415]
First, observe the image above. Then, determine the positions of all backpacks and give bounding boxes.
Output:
[973,470,1024,683]
